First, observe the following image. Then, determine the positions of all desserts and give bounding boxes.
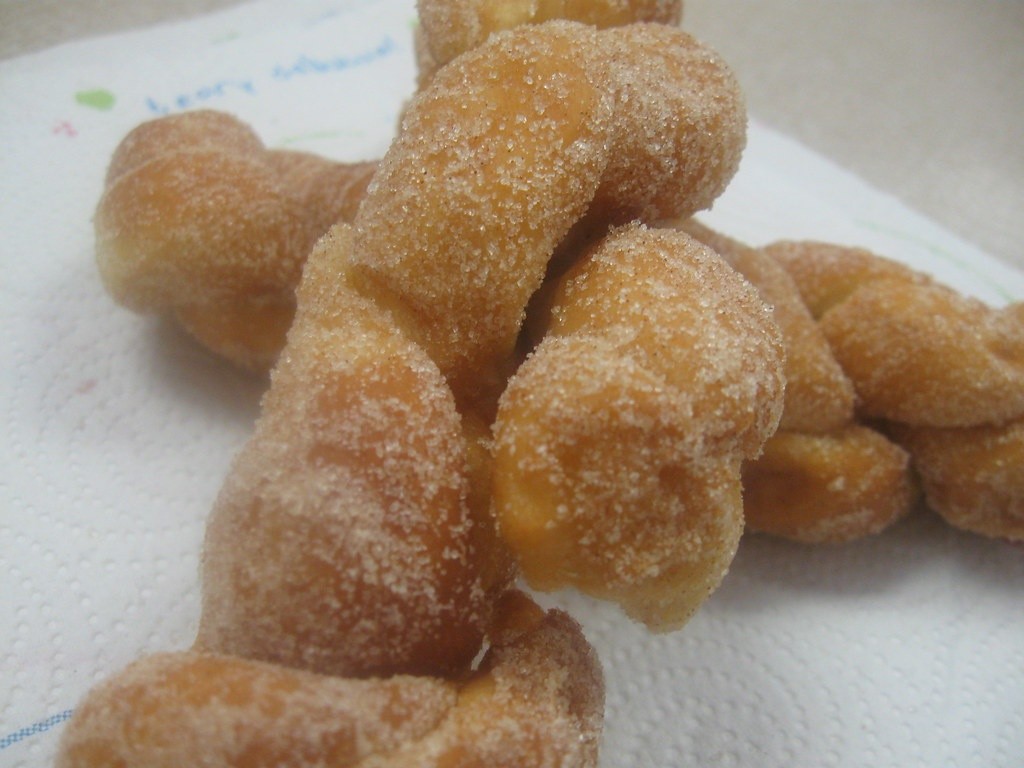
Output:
[50,0,1024,765]
